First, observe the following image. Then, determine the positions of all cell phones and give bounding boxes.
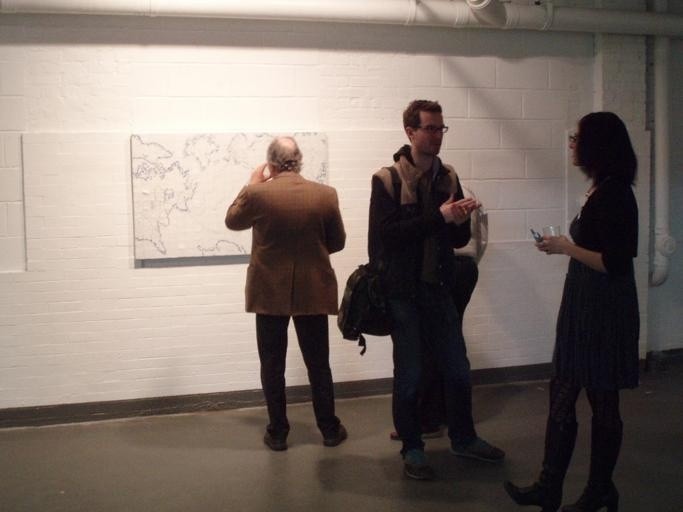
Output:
[534,232,543,242]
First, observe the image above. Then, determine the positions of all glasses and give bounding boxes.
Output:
[416,125,448,134]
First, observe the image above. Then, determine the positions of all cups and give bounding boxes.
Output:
[542,225,560,239]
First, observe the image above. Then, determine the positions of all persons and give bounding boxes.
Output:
[386,161,490,441]
[222,135,350,451]
[500,111,640,510]
[365,99,505,481]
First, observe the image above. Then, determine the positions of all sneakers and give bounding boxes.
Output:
[391,423,441,439]
[402,449,433,479]
[449,439,505,463]
[324,425,347,446]
[264,430,288,450]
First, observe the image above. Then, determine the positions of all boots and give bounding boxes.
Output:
[561,417,624,511]
[505,419,577,512]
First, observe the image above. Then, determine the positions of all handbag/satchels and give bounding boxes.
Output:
[337,265,394,341]
